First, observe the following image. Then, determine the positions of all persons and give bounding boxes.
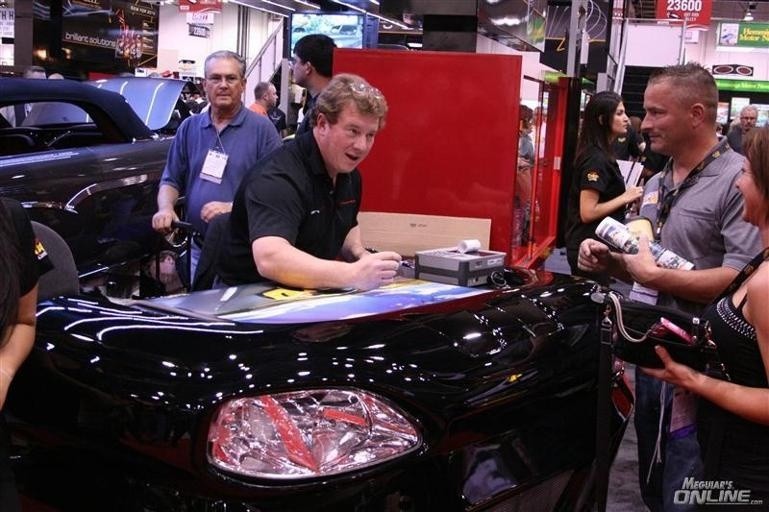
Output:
[512,105,546,247]
[0,66,47,127]
[186,94,199,109]
[576,64,769,512]
[212,73,402,291]
[152,50,283,290]
[292,34,336,108]
[564,91,643,290]
[0,196,53,411]
[249,81,289,138]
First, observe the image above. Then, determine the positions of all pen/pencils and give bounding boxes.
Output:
[366,248,415,269]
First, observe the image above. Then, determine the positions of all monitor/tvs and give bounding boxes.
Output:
[287,10,365,61]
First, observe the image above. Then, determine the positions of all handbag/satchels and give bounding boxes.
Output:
[608,291,708,374]
